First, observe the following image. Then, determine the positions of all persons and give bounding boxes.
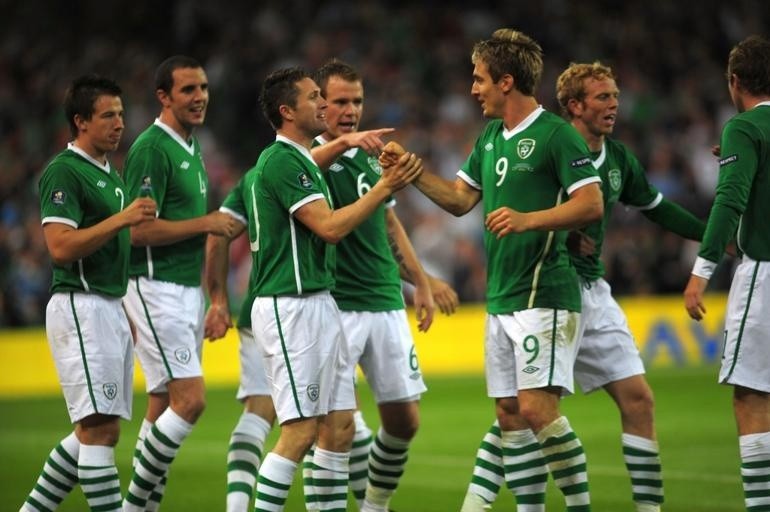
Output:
[205,64,459,512]
[0,0,769,325]
[462,64,707,511]
[121,56,241,512]
[378,28,604,511]
[683,35,770,511]
[18,75,155,511]
